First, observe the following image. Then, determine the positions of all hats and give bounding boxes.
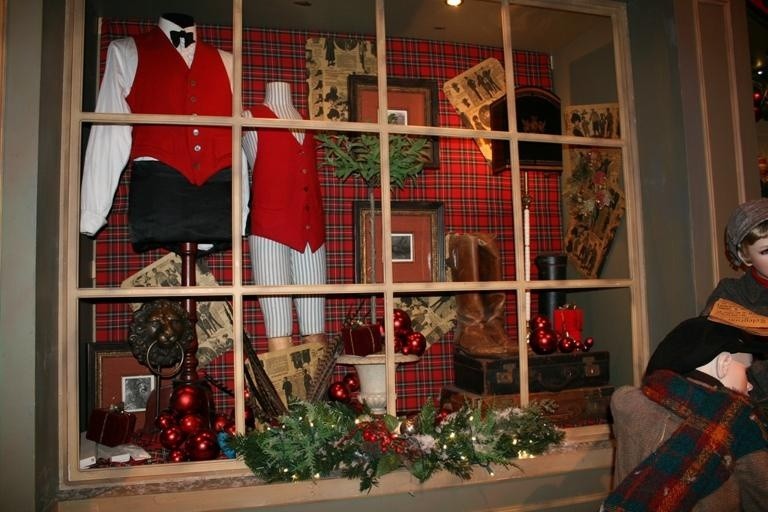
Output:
[723,197,768,269]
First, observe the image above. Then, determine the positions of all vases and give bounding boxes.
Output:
[333,352,420,420]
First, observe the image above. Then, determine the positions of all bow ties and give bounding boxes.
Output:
[168,29,195,48]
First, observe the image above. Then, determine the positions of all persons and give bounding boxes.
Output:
[79,14,253,251]
[222,333,233,350]
[168,259,181,275]
[240,80,330,351]
[474,72,493,98]
[703,198,768,322]
[282,376,292,404]
[152,267,171,287]
[480,67,501,93]
[196,312,212,339]
[303,369,313,391]
[198,301,226,330]
[164,269,180,285]
[472,116,492,149]
[201,315,216,335]
[464,77,482,101]
[639,314,768,458]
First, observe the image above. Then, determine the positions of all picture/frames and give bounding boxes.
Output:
[343,71,441,171]
[85,339,174,438]
[349,196,447,298]
[550,102,628,281]
